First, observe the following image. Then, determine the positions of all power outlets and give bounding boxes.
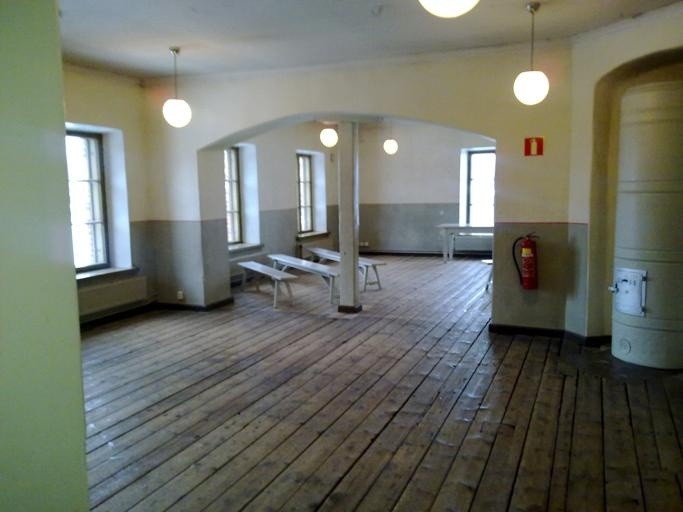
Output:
[176,290,184,301]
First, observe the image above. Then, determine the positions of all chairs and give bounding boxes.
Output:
[480,259,493,290]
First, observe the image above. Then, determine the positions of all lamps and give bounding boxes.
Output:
[512,4,553,107]
[160,48,193,130]
[384,138,399,155]
[418,1,481,20]
[320,127,339,149]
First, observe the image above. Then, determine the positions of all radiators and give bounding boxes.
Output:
[299,238,334,259]
[229,251,268,278]
[76,275,150,317]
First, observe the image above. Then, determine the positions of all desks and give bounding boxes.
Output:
[438,222,494,260]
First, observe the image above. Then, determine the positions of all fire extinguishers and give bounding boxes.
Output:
[512,231,540,290]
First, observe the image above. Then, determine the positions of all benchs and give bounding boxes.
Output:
[236,248,386,308]
